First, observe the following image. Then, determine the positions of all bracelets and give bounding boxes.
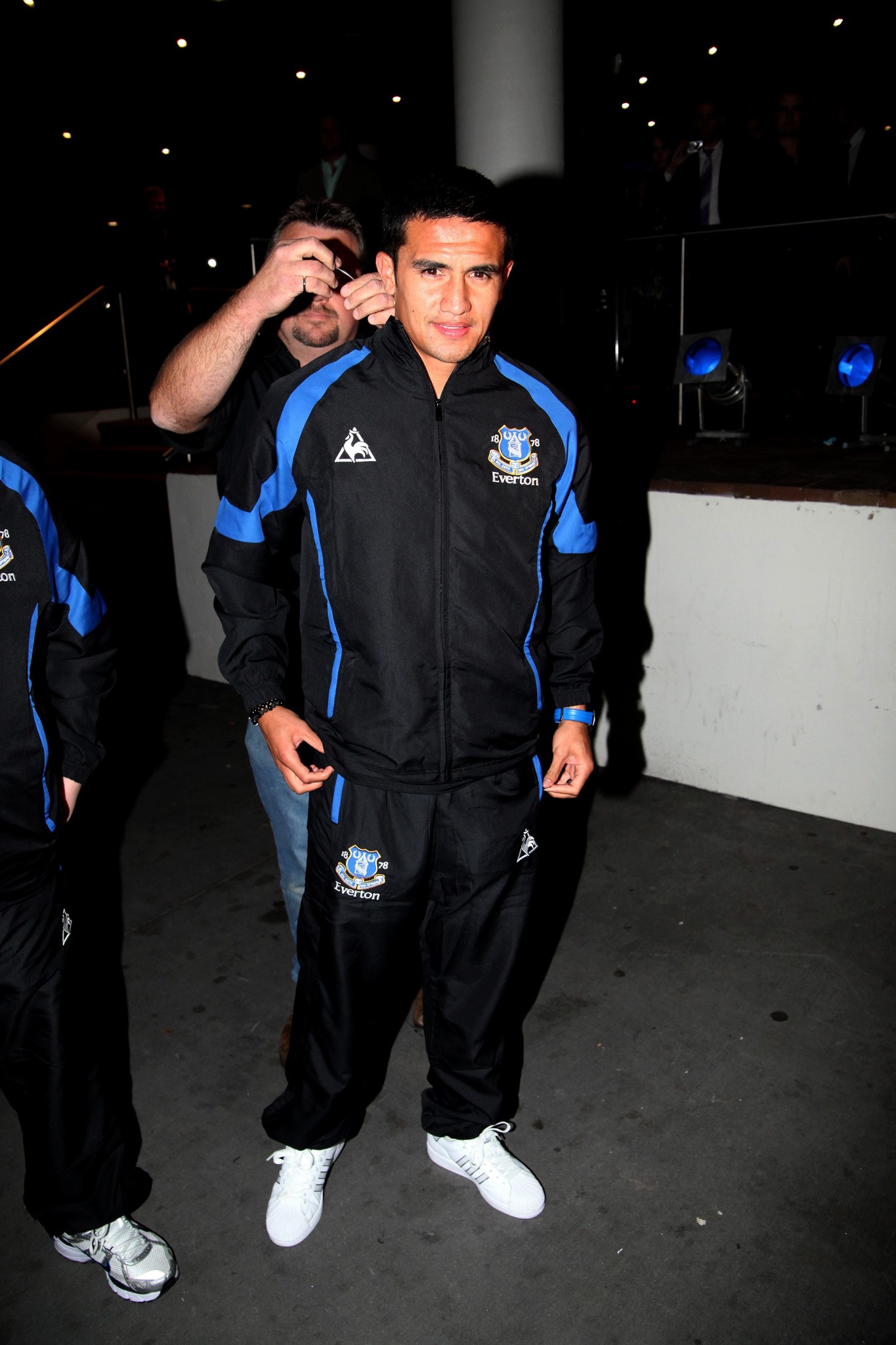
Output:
[249,699,284,726]
[555,708,596,728]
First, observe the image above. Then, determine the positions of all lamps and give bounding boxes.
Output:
[675,328,752,438]
[825,332,896,447]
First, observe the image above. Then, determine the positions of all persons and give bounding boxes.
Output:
[0,441,182,1303]
[204,169,620,1249]
[154,190,435,1066]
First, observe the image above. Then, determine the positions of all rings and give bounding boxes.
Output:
[303,276,306,291]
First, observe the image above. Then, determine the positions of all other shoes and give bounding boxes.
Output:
[414,988,424,1030]
[279,1012,294,1065]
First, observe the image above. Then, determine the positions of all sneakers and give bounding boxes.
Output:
[426,1121,545,1219]
[266,1141,345,1247]
[54,1215,179,1302]
